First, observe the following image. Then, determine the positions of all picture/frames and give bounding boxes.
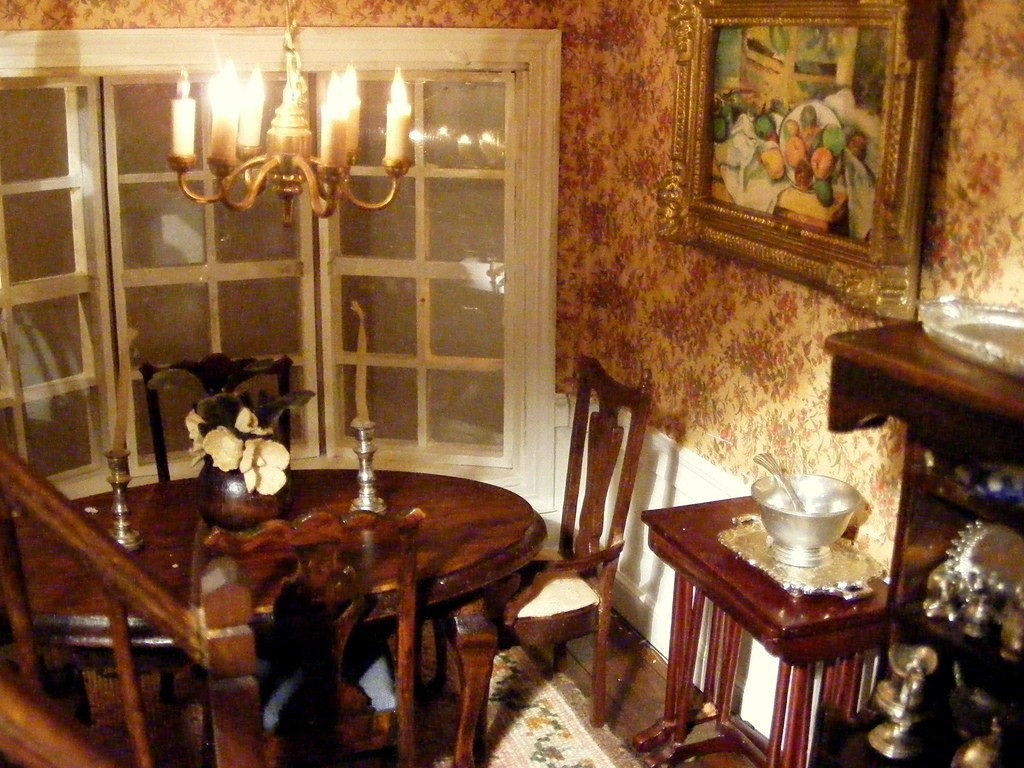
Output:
[656,0,941,321]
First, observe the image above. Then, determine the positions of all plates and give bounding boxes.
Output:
[919,296,1024,380]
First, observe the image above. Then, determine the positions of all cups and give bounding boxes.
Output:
[873,641,939,718]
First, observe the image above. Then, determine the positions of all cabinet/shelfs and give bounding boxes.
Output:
[807,322,1024,768]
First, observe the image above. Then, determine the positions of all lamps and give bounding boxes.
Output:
[168,1,410,228]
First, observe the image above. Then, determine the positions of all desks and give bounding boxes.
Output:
[1,469,548,768]
[632,497,888,768]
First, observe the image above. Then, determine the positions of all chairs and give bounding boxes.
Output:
[205,507,426,768]
[429,354,654,764]
[139,352,295,701]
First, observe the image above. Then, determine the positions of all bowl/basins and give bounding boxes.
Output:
[752,473,862,569]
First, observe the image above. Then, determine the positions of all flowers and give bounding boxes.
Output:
[185,359,315,495]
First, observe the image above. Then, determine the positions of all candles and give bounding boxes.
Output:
[351,301,370,425]
[113,329,138,453]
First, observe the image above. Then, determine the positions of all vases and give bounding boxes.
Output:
[195,454,295,530]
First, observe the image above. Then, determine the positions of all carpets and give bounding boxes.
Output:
[1,641,645,768]
